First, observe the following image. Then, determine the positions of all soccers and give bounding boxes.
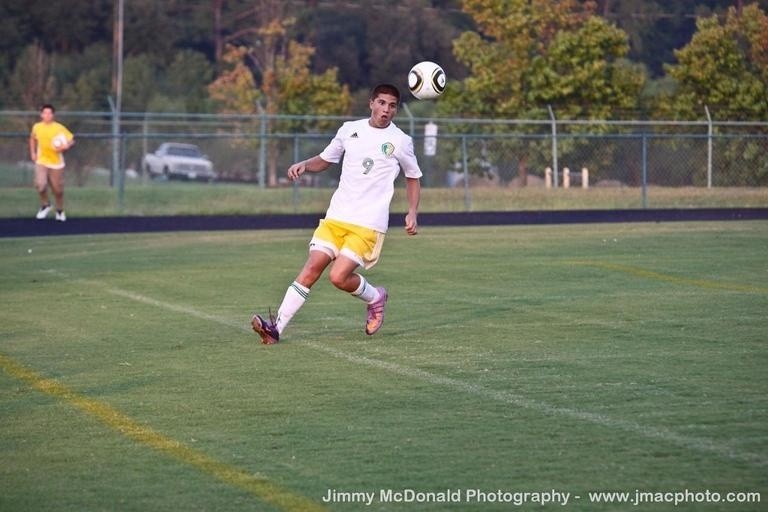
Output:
[407,62,446,100]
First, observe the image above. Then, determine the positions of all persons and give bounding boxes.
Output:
[252,83,423,345]
[29,104,75,223]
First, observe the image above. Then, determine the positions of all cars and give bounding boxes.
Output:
[141,142,214,181]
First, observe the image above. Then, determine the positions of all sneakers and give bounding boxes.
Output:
[250,314,279,345]
[366,287,388,336]
[55,208,66,221]
[36,202,52,220]
